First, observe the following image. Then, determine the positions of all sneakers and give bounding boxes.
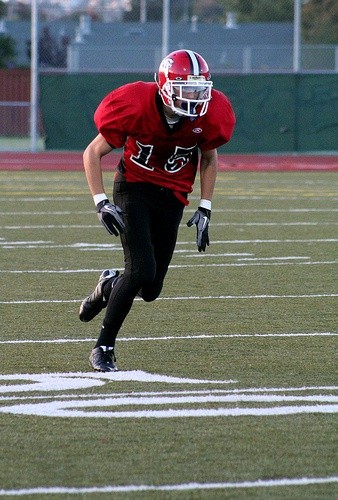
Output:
[79,269,120,322]
[89,345,119,372]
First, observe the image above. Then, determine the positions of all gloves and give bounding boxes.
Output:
[187,198,211,253]
[93,192,126,237]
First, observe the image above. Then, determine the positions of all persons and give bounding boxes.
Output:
[34,27,57,65]
[79,50,236,371]
[53,36,70,67]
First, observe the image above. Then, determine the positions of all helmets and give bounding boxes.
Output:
[154,49,213,117]
